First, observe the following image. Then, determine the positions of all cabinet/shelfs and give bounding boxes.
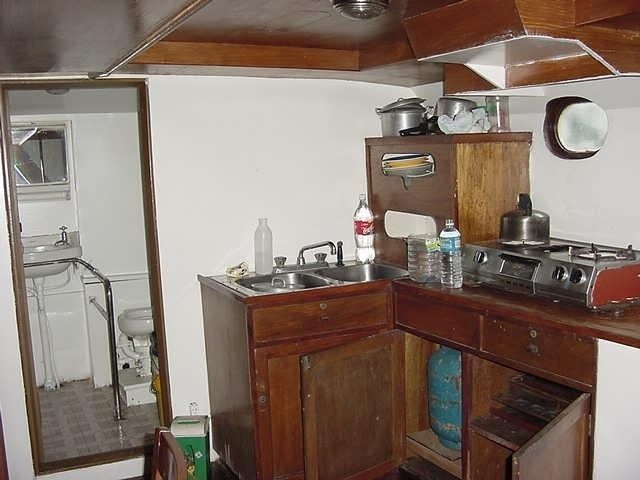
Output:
[197,275,397,480]
[393,279,596,480]
[365,132,533,271]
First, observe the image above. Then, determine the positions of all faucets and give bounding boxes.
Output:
[295,241,338,268]
[54,225,72,248]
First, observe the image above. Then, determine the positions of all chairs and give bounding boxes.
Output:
[125,427,187,480]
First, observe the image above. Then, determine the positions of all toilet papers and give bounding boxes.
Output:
[385,209,438,242]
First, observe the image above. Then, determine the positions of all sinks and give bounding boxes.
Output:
[225,266,332,296]
[20,243,83,280]
[117,306,155,339]
[303,258,409,285]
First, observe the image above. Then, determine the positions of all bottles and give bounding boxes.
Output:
[253,216,275,276]
[439,217,465,289]
[353,193,377,264]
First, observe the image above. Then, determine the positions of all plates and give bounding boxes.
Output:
[382,155,434,175]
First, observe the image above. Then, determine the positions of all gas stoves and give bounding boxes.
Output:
[460,235,639,310]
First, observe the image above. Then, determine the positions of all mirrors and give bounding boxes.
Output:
[10,121,76,187]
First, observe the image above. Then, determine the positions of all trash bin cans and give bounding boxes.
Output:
[170,415,211,480]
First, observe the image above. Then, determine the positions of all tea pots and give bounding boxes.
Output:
[497,191,552,246]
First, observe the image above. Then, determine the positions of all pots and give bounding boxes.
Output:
[372,96,478,137]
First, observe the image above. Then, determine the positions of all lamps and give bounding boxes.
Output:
[332,0,391,21]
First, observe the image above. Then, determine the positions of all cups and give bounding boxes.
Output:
[485,96,511,134]
[406,233,439,283]
